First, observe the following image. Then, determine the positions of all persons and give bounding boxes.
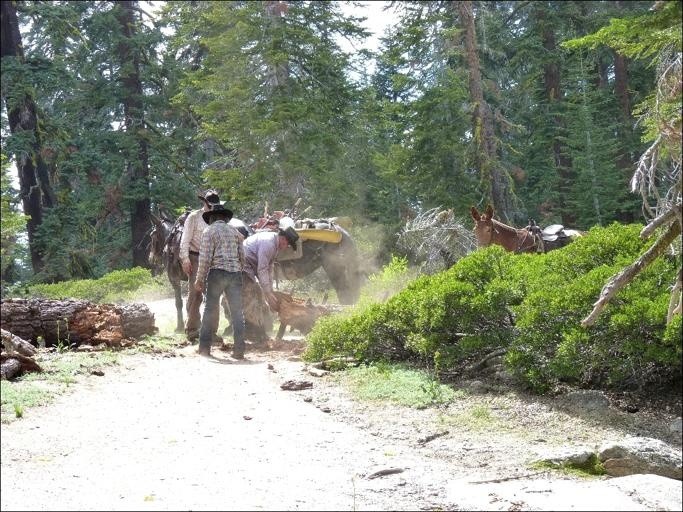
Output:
[192,204,245,359]
[242,225,298,348]
[176,190,221,342]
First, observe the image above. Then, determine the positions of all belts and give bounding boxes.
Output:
[189,251,198,256]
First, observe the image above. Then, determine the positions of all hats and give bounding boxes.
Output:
[202,204,232,225]
[197,190,227,206]
[278,226,299,252]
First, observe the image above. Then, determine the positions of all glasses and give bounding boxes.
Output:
[205,201,212,209]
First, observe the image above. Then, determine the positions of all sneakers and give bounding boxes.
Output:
[194,345,211,355]
[231,351,244,359]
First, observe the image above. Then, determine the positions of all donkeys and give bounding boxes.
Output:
[147,207,255,335]
[255,222,360,306]
[469,205,582,256]
